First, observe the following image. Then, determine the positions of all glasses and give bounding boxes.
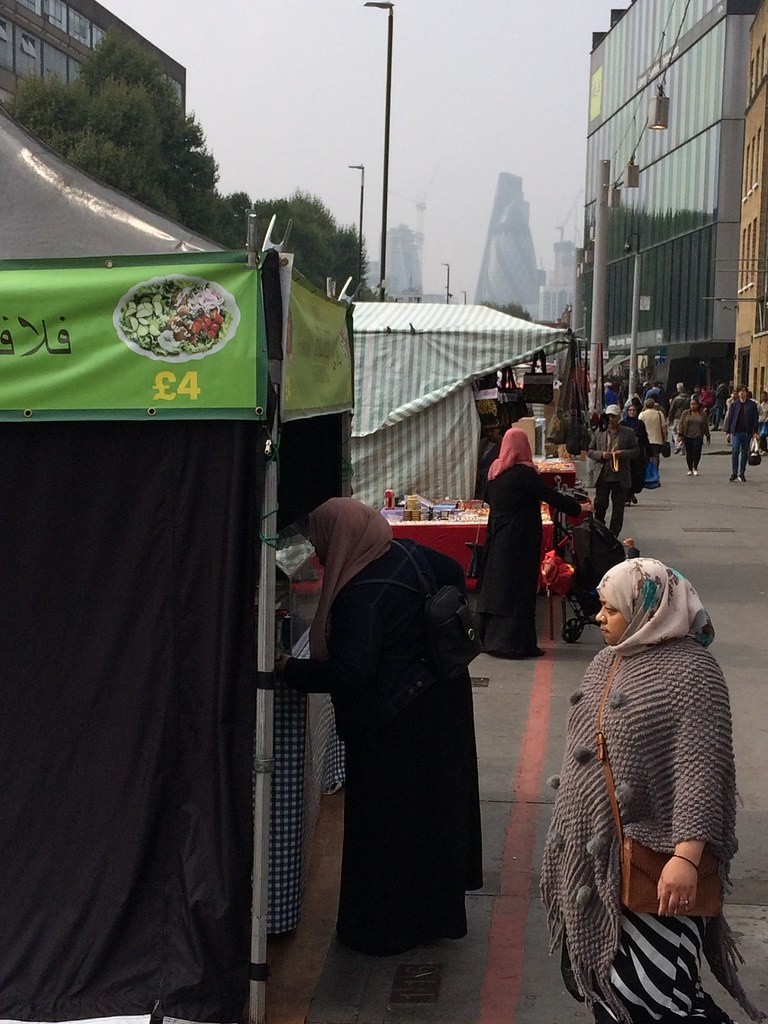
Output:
[627,409,636,412]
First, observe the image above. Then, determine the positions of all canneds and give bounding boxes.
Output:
[383,489,396,510]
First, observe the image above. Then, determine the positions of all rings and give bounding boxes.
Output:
[680,899,688,904]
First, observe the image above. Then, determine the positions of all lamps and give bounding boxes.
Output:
[626,161,639,187]
[649,88,670,129]
[608,186,620,207]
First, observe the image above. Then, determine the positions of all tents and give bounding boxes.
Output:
[0,107,352,1024]
[347,301,574,512]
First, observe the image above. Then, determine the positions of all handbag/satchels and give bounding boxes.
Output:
[759,422,768,437]
[662,442,671,458]
[621,837,722,918]
[747,437,762,467]
[464,542,484,578]
[644,460,662,489]
[473,348,593,456]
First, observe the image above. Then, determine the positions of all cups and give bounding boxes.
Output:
[383,489,396,510]
[403,510,412,521]
[411,511,420,521]
[406,496,418,510]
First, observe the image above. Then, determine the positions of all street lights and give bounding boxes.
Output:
[623,233,642,396]
[348,163,365,297]
[363,1,393,303]
[461,292,466,304]
[441,262,450,302]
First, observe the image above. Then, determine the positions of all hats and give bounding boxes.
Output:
[604,404,620,416]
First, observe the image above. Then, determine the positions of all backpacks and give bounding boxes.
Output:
[538,533,577,598]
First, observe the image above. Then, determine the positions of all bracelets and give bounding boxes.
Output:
[672,854,698,870]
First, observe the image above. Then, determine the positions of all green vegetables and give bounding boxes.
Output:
[120,278,232,357]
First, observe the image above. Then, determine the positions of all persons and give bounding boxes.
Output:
[271,497,481,957]
[474,428,594,659]
[603,375,768,456]
[677,399,711,475]
[725,385,759,483]
[587,404,640,539]
[475,418,502,503]
[637,398,667,469]
[618,405,654,506]
[540,556,737,1024]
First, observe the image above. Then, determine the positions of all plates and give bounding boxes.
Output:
[112,273,241,363]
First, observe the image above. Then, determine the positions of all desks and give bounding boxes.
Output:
[531,454,575,490]
[386,502,555,595]
[565,500,591,528]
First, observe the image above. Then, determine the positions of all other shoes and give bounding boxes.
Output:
[630,493,638,504]
[693,468,698,476]
[526,648,546,657]
[710,426,718,431]
[674,446,681,454]
[625,496,631,506]
[687,471,693,475]
[679,450,685,455]
[728,474,738,482]
[737,474,746,483]
[759,450,768,456]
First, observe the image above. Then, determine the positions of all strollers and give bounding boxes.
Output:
[552,506,627,644]
